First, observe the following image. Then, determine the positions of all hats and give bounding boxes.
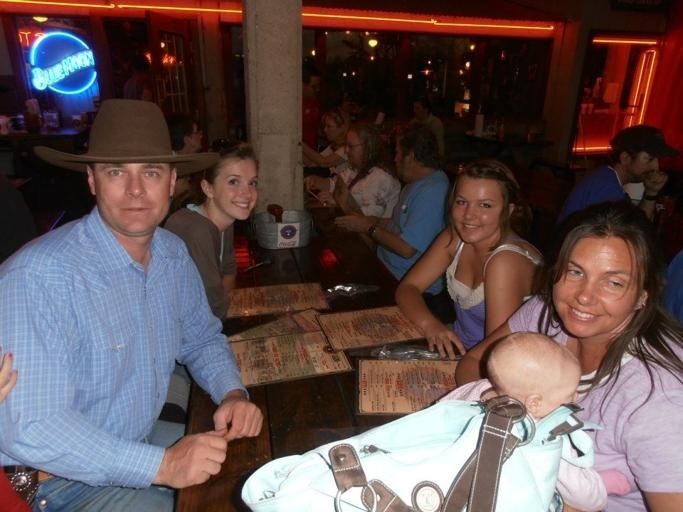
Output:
[34,99,221,179]
[609,123,682,159]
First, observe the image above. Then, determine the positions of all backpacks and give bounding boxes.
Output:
[238,396,571,512]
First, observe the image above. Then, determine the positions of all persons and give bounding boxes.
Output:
[300,61,320,148]
[120,51,154,100]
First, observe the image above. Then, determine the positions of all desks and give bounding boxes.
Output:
[171,206,467,508]
